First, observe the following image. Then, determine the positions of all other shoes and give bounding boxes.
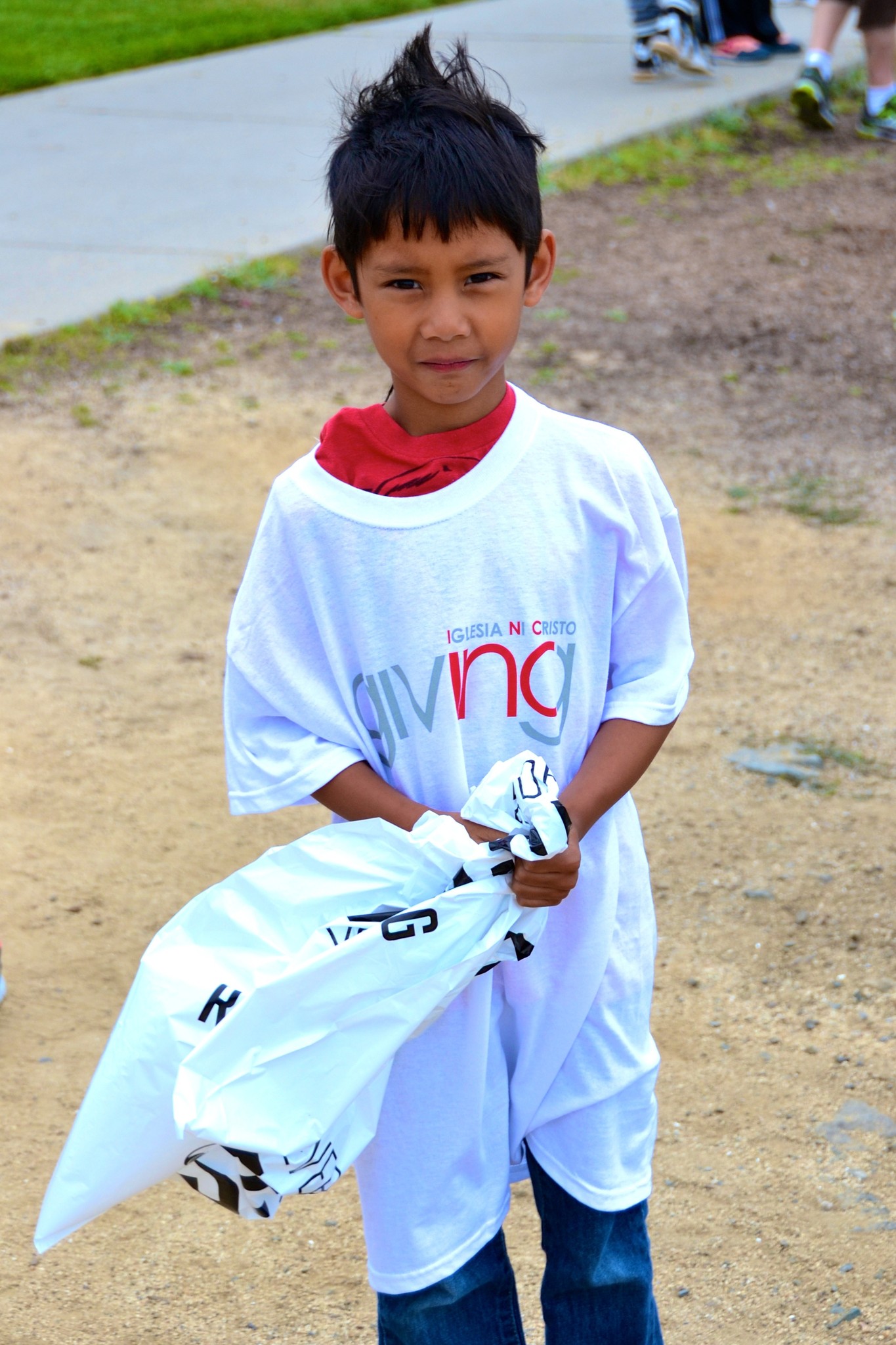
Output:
[636,60,662,83]
[763,36,803,55]
[651,32,709,78]
[712,35,770,65]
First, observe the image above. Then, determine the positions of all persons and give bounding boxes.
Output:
[220,25,696,1345]
[791,0,896,142]
[628,1,801,83]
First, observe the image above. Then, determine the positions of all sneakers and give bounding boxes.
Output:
[792,69,838,134]
[853,95,896,143]
